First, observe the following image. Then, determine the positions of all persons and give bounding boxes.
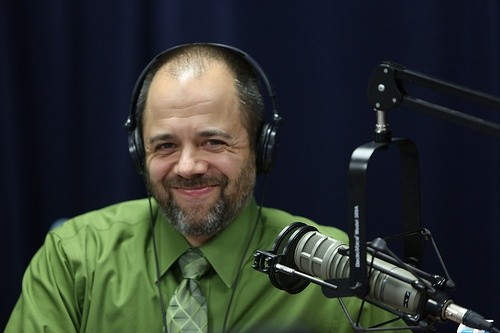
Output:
[1,42,427,332]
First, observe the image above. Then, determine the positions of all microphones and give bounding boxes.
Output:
[298,232,453,323]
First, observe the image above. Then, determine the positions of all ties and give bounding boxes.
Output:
[162,249,213,333]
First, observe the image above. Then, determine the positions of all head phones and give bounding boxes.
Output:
[124,42,285,177]
